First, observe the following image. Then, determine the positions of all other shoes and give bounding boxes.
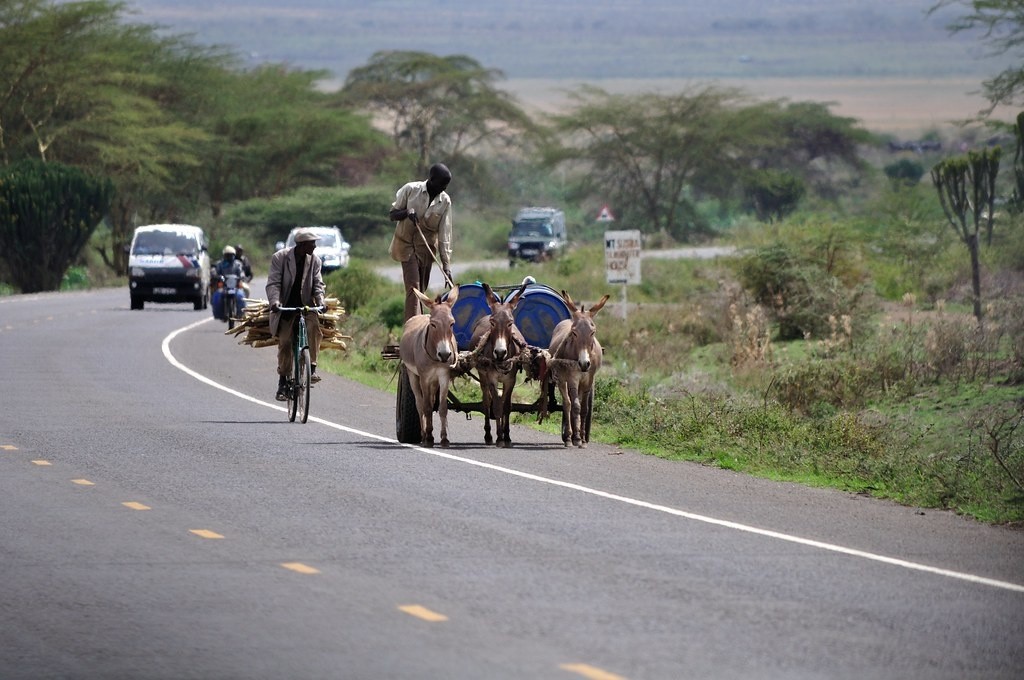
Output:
[276,380,288,401]
[311,371,320,384]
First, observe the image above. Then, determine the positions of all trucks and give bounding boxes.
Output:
[507,206,568,268]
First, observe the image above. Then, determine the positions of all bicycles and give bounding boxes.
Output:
[276,305,325,424]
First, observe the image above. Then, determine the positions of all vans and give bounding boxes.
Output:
[123,223,211,310]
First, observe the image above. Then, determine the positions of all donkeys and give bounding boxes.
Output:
[399,282,460,449]
[470,283,529,449]
[550,290,611,450]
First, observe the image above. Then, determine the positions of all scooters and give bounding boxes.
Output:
[215,274,249,330]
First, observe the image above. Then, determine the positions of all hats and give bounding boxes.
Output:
[294,229,322,242]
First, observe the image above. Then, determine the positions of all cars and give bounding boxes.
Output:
[276,226,351,274]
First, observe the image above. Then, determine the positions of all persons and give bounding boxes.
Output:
[210,243,253,300]
[389,162,452,323]
[265,229,326,401]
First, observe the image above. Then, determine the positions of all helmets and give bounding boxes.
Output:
[223,245,235,256]
[235,244,244,251]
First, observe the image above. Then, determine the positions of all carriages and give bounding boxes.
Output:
[379,282,610,450]
